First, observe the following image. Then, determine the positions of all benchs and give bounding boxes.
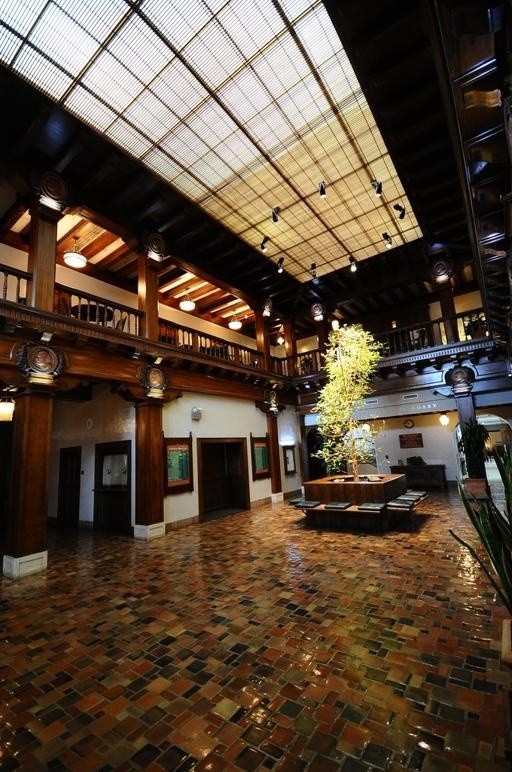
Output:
[289,489,428,514]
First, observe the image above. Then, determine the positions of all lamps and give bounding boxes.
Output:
[63,235,87,268]
[179,288,195,311]
[228,310,242,329]
[438,412,449,426]
[0,393,16,422]
[260,181,404,285]
[191,407,202,420]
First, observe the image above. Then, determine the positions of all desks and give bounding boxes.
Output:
[71,304,113,326]
[389,465,446,489]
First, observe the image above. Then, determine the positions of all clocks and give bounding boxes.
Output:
[404,419,414,428]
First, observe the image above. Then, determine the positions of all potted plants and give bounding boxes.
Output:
[455,416,491,499]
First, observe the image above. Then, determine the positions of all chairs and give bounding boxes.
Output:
[61,296,83,319]
[108,315,127,332]
